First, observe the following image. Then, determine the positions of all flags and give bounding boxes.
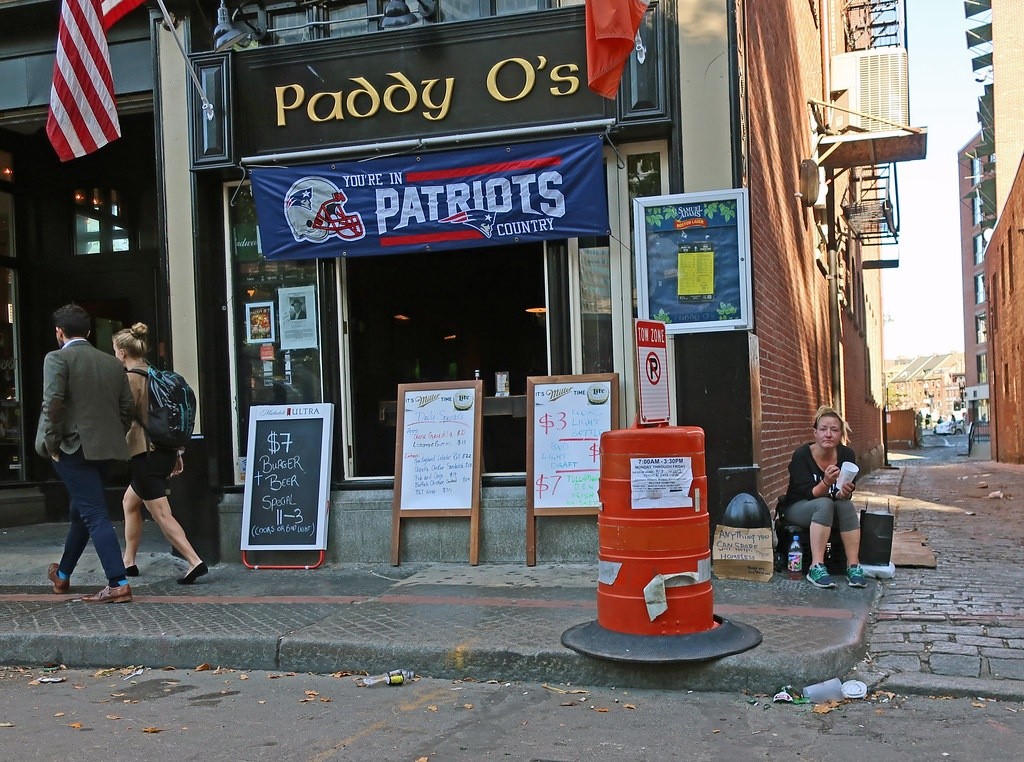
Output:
[584,0,649,99]
[46,1,145,160]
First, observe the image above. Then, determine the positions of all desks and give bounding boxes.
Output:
[370,392,528,430]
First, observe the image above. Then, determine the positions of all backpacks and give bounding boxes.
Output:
[126,368,199,448]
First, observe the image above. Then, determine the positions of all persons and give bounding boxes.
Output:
[291,299,306,320]
[112,323,208,584]
[782,406,870,588]
[34,304,137,603]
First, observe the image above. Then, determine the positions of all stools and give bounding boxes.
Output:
[775,513,854,571]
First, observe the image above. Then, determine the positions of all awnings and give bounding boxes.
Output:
[807,98,928,185]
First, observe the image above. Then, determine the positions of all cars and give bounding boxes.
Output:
[934,419,966,435]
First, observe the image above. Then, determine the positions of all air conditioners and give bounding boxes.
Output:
[829,44,914,135]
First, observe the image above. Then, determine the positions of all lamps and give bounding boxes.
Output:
[378,0,441,29]
[799,155,824,209]
[212,0,268,56]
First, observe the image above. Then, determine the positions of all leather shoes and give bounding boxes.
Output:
[47,562,70,593]
[82,586,133,605]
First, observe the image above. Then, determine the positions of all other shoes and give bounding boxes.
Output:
[176,562,208,585]
[125,565,140,577]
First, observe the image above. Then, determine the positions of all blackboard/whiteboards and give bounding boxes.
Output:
[526,375,620,516]
[237,402,333,551]
[395,381,482,518]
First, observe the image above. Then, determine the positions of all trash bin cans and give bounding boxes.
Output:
[599,424,719,635]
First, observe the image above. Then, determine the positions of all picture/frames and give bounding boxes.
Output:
[244,300,278,344]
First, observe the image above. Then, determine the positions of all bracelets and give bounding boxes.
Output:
[822,479,832,487]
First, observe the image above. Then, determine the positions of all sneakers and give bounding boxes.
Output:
[807,562,837,589]
[844,563,868,587]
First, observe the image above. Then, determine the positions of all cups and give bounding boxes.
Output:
[803,677,844,703]
[836,462,859,490]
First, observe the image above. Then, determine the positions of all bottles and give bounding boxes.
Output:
[475,369,480,380]
[824,542,835,574]
[787,536,803,573]
[363,669,415,688]
